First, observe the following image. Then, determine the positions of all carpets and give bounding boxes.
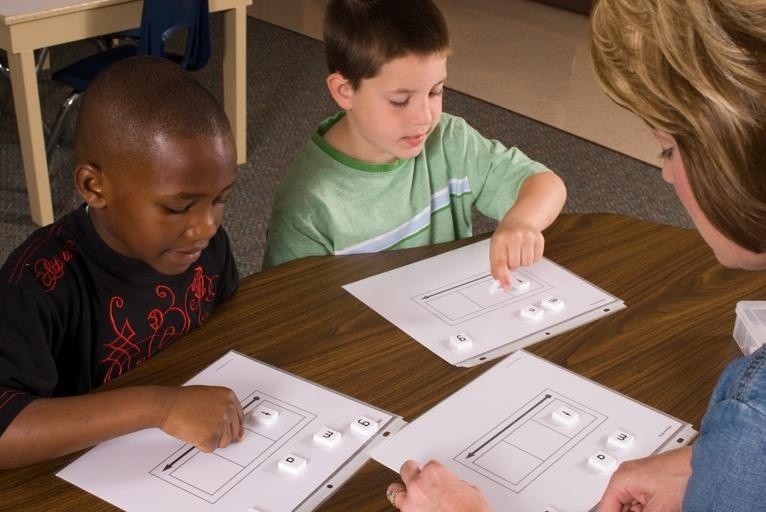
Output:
[246,1,664,168]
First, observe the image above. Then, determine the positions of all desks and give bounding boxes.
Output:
[0,212,766,512]
[1,0,254,228]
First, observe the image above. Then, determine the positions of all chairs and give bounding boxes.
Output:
[46,0,211,154]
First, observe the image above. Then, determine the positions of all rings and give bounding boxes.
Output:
[386,486,407,507]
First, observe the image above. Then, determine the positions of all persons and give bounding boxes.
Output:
[0,52,247,470]
[262,0,569,291]
[378,1,766,512]
